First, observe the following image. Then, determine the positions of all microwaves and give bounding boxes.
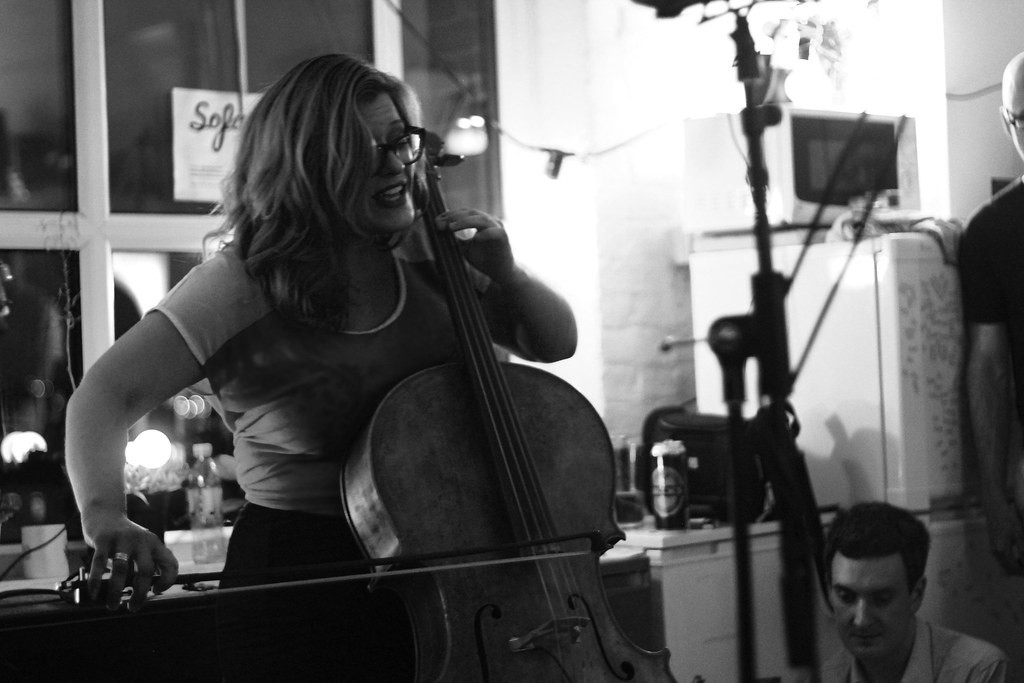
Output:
[686,107,921,233]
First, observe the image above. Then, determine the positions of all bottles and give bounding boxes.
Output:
[186,443,226,563]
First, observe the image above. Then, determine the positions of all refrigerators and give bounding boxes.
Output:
[691,235,1024,683]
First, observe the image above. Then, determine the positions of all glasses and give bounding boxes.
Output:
[1003,107,1024,129]
[362,125,426,178]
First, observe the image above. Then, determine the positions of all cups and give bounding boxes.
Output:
[21,524,69,579]
[612,435,644,529]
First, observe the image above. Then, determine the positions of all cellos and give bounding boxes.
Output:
[337,129,679,683]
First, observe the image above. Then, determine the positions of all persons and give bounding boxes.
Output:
[65,51,578,683]
[822,501,1011,683]
[961,52,1023,575]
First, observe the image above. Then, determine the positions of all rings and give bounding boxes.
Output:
[113,552,130,562]
